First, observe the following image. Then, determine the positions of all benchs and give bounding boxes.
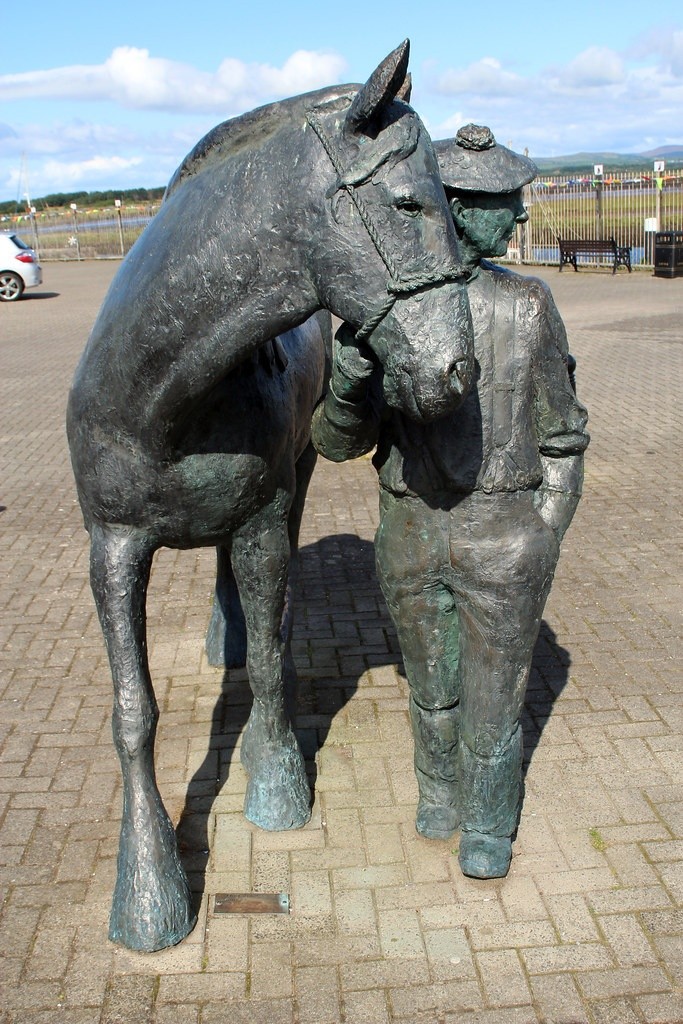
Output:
[557,237,632,275]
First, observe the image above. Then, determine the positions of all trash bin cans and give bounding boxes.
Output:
[651,230,683,279]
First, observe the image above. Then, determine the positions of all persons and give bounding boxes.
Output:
[313,123,589,878]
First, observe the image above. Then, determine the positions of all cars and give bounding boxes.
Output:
[0,234,45,301]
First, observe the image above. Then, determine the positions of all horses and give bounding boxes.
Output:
[65,38,478,957]
